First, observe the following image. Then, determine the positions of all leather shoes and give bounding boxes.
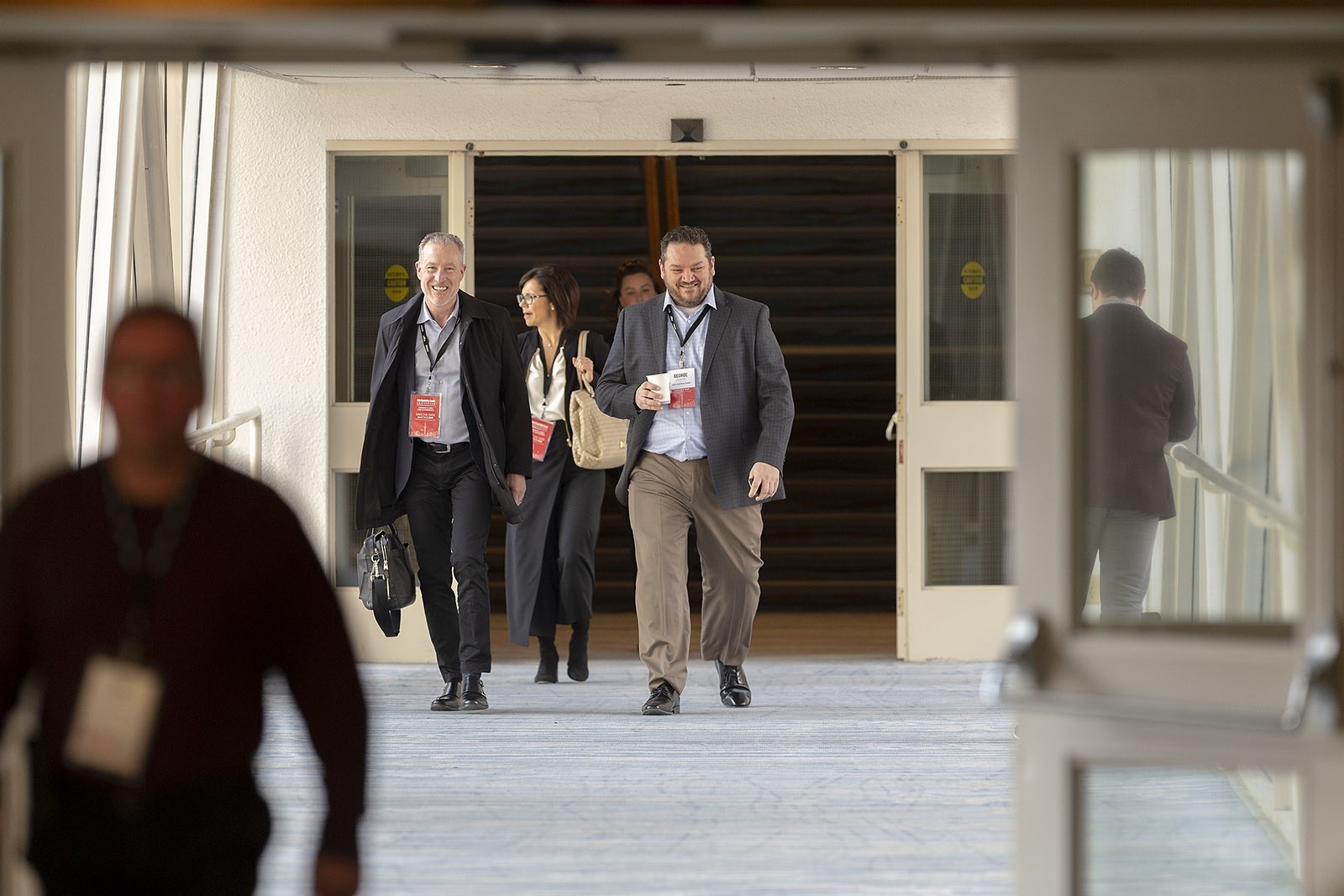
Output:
[462,677,490,711]
[430,680,460,711]
[641,685,680,716]
[715,658,752,707]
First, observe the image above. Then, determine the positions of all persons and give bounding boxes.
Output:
[0,302,368,896]
[504,266,610,684]
[1073,248,1197,630]
[354,233,532,711]
[595,225,795,714]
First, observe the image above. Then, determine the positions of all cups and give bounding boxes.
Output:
[646,372,671,404]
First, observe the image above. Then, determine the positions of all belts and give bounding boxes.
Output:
[416,437,471,454]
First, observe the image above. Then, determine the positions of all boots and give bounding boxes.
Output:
[566,625,590,682]
[534,636,560,684]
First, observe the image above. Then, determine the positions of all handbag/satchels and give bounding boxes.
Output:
[570,329,628,470]
[353,522,416,636]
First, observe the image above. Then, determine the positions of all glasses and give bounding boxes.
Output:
[516,294,550,304]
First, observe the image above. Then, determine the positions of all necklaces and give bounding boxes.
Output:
[542,337,558,347]
